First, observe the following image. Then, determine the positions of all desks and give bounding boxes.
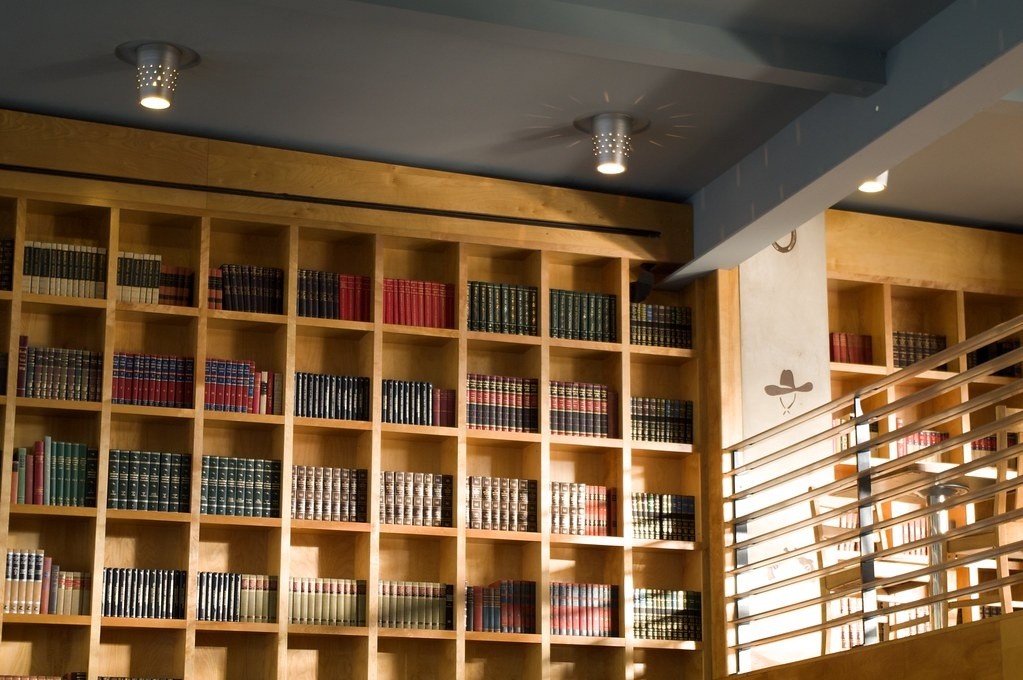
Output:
[830,462,1018,631]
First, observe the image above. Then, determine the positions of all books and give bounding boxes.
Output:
[0,237,704,680]
[827,332,1022,649]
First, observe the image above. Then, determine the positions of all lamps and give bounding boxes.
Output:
[137,46,180,112]
[593,113,636,174]
[857,166,889,192]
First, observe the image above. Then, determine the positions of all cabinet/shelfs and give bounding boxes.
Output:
[827,278,1023,624]
[0,168,714,680]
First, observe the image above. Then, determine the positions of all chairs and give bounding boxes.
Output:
[947,406,1023,622]
[809,486,931,655]
